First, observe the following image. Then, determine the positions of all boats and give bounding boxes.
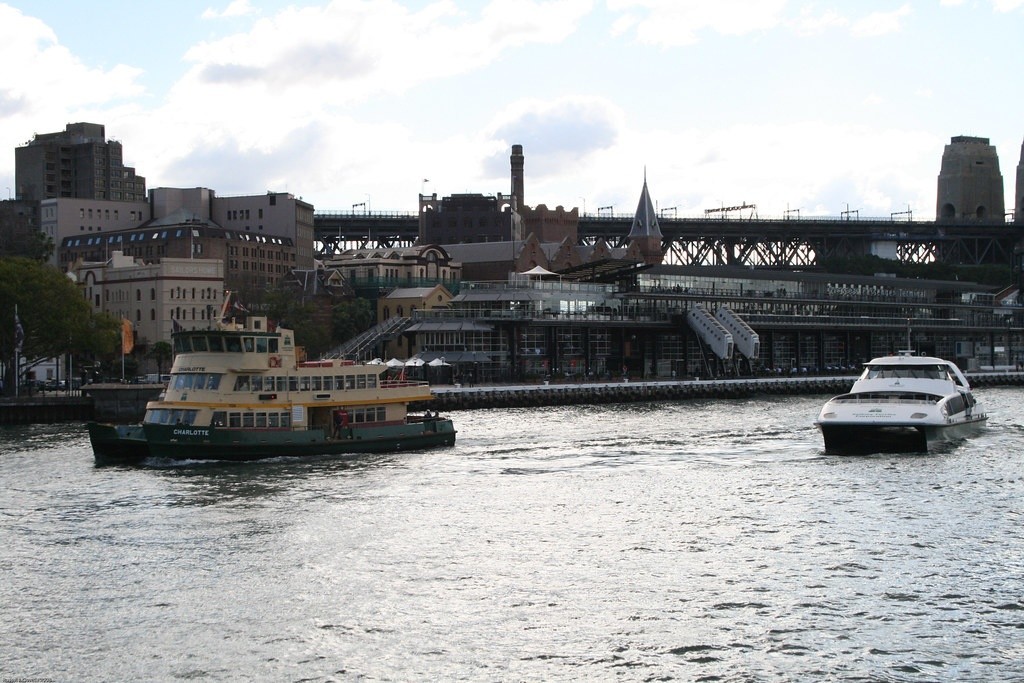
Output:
[817,317,989,452]
[87,290,459,460]
